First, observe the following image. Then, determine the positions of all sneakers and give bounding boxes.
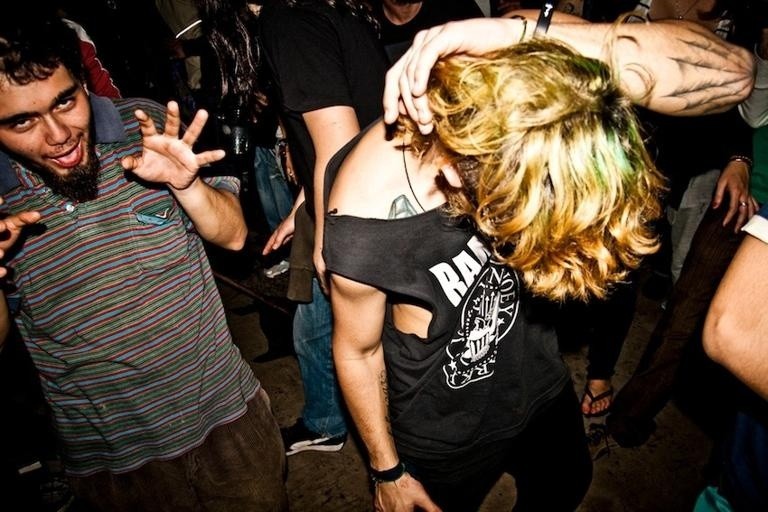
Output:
[281,418,348,457]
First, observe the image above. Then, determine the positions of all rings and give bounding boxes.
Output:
[738,201,749,208]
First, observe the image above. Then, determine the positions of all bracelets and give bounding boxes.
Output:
[512,0,556,42]
[367,461,407,484]
[727,155,753,166]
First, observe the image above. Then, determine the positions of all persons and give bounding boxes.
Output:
[320,20,756,512]
[1,13,290,509]
[0,0,768,466]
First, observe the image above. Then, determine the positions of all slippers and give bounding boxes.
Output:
[579,380,615,417]
